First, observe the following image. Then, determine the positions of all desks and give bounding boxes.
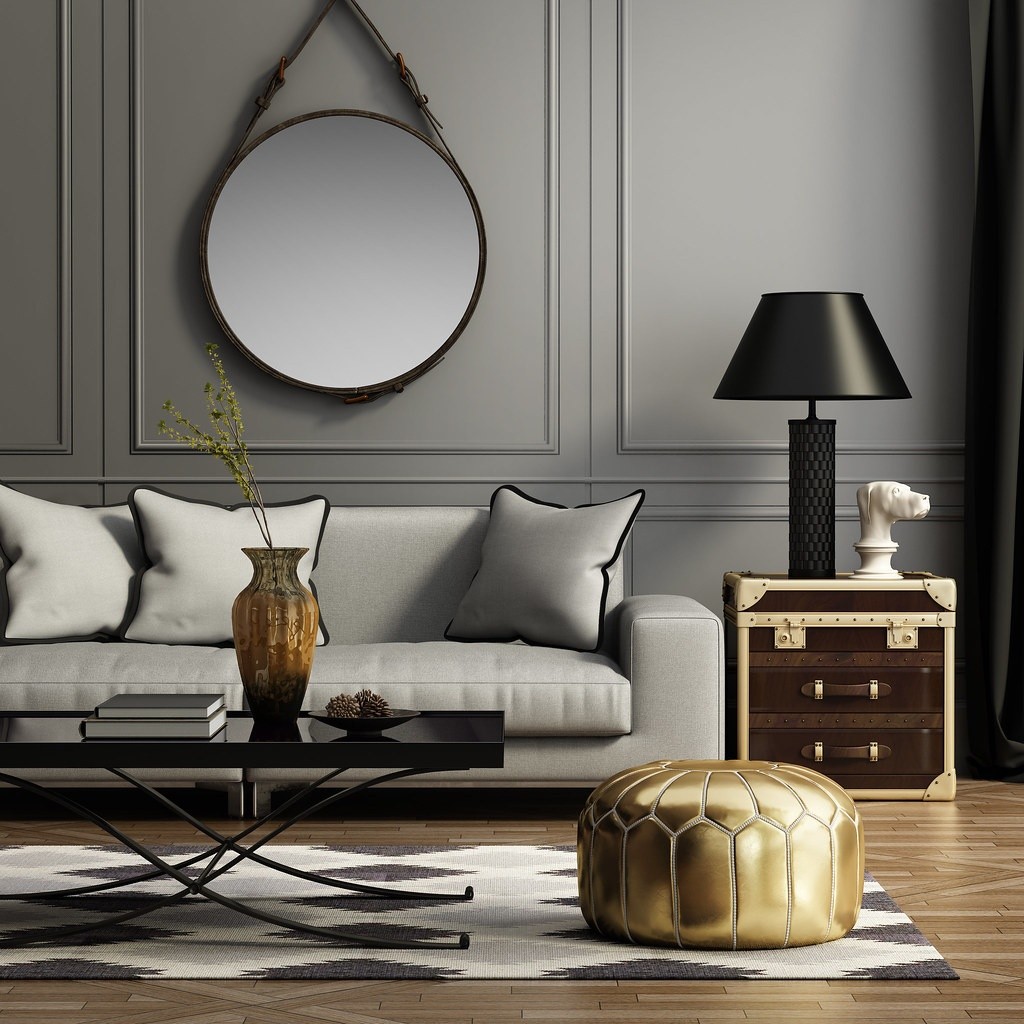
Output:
[0,710,505,951]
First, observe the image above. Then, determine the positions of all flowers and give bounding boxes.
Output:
[156,341,297,548]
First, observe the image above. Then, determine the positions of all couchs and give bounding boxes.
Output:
[0,505,724,817]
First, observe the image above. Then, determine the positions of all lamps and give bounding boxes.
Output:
[713,293,913,581]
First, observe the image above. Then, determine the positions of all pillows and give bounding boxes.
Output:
[445,484,646,651]
[119,485,330,646]
[0,483,140,642]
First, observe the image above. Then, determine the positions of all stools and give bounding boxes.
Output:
[574,758,864,948]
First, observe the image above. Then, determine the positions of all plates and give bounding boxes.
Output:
[308,710,421,730]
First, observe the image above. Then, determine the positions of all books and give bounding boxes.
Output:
[78,694,228,740]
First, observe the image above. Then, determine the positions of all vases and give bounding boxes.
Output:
[231,548,320,726]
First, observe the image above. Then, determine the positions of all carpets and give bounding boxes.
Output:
[0,845,961,980]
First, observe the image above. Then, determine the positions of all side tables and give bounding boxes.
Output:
[723,571,957,801]
